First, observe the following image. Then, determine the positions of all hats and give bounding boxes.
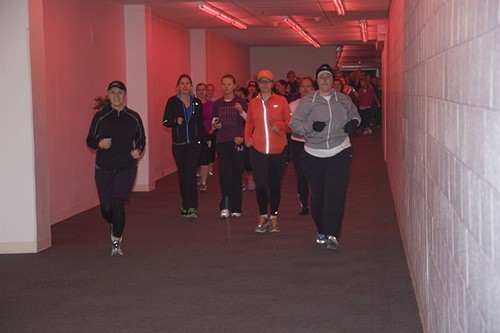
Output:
[256,69,273,81]
[108,80,126,91]
[316,63,334,76]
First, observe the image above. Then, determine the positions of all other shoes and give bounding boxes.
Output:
[255,214,272,233]
[207,163,215,176]
[357,125,381,135]
[220,208,230,218]
[298,207,310,216]
[269,217,281,233]
[241,174,247,193]
[326,236,338,250]
[196,172,202,186]
[231,212,242,217]
[187,207,197,218]
[180,208,189,218]
[246,172,256,191]
[199,183,209,193]
[316,233,325,244]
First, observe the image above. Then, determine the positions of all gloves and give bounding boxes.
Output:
[312,120,326,132]
[343,118,359,134]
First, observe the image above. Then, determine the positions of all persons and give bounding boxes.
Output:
[288,63,361,249]
[86,80,146,256]
[197,65,382,216]
[211,74,248,218]
[163,74,204,218]
[244,69,292,233]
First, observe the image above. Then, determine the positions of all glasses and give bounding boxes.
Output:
[258,79,272,84]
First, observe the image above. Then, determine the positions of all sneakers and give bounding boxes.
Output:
[110,241,124,256]
[110,221,123,245]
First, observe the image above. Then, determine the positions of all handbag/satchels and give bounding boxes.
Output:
[194,138,216,166]
